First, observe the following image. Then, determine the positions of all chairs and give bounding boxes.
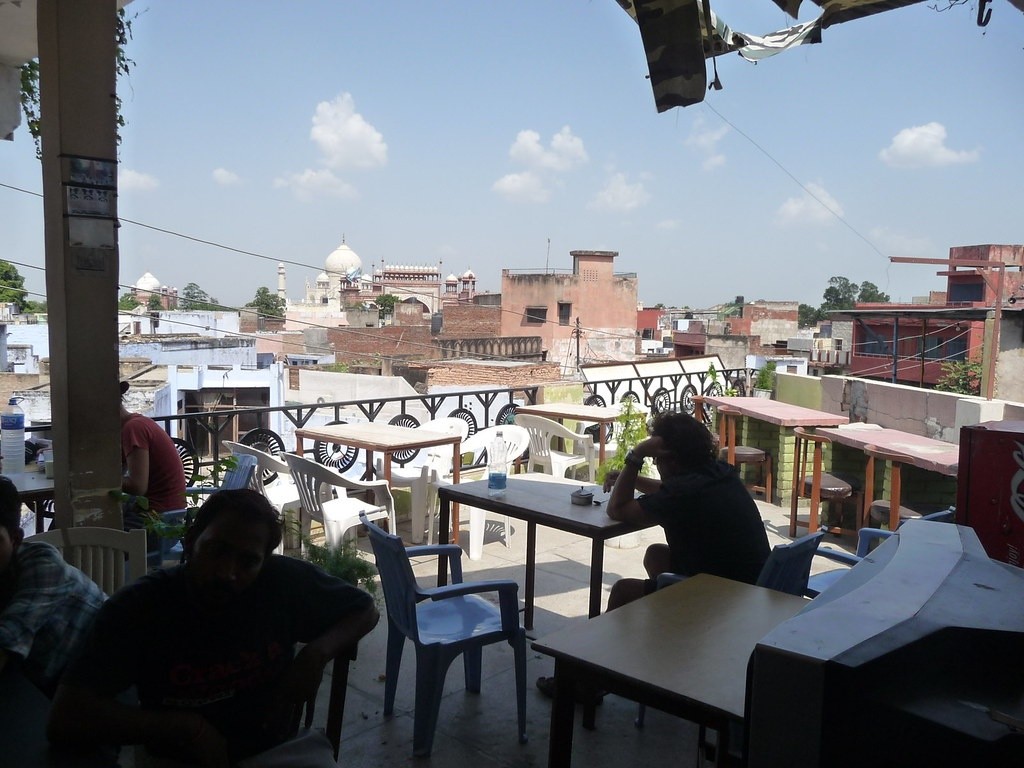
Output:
[22,403,955,757]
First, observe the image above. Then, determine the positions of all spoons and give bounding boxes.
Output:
[593,500,608,505]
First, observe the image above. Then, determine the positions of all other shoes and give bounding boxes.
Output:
[537,676,608,706]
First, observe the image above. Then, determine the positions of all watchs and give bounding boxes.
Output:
[624,447,645,470]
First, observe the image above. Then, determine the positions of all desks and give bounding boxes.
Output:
[706,397,849,511]
[518,403,648,465]
[815,428,960,544]
[532,571,810,768]
[295,423,461,547]
[439,472,659,630]
[0,472,55,535]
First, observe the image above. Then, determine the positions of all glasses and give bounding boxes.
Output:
[653,457,664,465]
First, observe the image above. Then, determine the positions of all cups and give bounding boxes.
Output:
[42,450,54,479]
[487,430,507,498]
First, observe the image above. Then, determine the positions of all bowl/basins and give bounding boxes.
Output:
[570,493,594,506]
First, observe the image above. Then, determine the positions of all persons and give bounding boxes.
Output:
[42,488,380,768]
[47,382,187,564]
[0,477,110,768]
[536,410,771,706]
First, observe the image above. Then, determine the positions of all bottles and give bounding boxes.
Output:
[1,397,25,475]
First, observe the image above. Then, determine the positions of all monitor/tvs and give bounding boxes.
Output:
[743,518,1024,768]
